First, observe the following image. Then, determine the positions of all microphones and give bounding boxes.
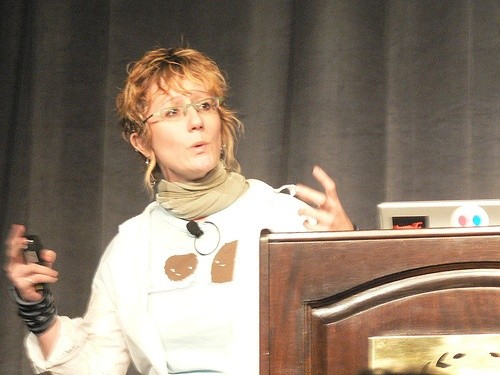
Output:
[186,220,204,238]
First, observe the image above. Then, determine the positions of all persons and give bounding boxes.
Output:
[5,44,362,375]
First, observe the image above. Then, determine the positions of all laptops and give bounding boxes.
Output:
[377,198,500,231]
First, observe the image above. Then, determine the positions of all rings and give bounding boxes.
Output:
[24,277,37,286]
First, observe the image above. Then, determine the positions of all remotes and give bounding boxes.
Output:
[23,235,46,292]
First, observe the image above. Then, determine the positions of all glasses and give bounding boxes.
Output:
[142,98,219,124]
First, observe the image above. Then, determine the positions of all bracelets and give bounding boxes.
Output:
[351,224,361,231]
[11,282,58,335]
